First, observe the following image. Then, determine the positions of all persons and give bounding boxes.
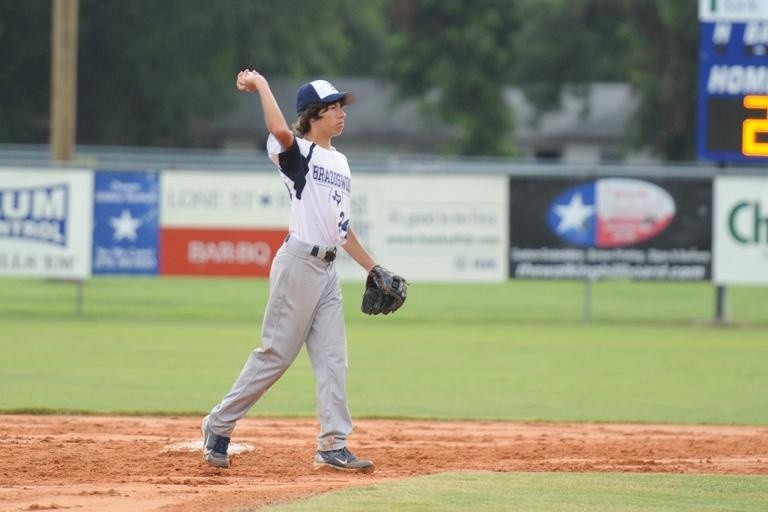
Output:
[200,69,407,472]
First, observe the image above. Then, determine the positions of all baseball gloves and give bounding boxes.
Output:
[361,266,407,315]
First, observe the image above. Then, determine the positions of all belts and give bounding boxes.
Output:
[285,236,337,261]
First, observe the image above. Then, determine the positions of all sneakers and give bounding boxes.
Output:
[201,416,230,468]
[314,447,375,474]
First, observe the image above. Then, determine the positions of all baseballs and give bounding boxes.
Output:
[240,71,253,79]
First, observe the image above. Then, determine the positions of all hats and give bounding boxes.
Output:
[297,80,356,112]
